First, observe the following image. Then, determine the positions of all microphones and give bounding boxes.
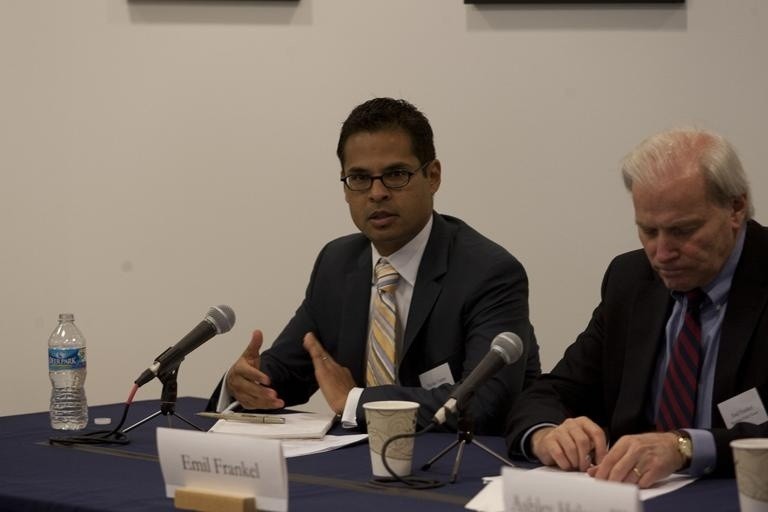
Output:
[431,332,523,425]
[134,305,236,387]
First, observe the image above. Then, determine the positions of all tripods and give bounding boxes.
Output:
[122,403,204,433]
[421,431,514,483]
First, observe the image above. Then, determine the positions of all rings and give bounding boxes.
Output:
[633,467,642,479]
[322,354,329,364]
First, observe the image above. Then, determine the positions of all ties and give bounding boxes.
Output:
[365,258,401,388]
[653,290,707,435]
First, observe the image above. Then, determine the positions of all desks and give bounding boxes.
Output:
[0,389,738,510]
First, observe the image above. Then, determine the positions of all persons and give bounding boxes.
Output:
[502,123,768,485]
[206,97,542,432]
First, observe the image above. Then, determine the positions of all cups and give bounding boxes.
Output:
[726,436,768,512]
[363,396,419,482]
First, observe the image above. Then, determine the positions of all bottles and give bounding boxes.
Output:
[45,310,89,433]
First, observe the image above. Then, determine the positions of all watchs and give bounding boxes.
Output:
[672,425,694,466]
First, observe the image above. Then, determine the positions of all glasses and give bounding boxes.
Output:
[340,160,431,193]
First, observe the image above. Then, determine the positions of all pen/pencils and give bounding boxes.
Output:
[194,409,289,425]
[584,446,600,462]
